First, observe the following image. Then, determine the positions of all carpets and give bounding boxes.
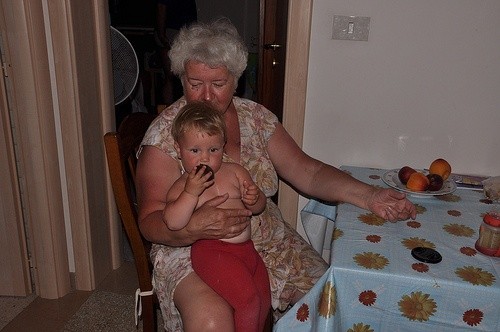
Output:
[64,289,165,332]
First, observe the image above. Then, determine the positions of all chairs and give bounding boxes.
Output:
[103,104,272,332]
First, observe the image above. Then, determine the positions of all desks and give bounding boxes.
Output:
[272,165,500,332]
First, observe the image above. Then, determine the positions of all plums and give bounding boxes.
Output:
[426,174,443,190]
[399,166,416,185]
[195,164,215,182]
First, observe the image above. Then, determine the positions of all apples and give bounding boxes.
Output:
[429,158,452,180]
[407,172,429,191]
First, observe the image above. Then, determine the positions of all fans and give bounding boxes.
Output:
[110,26,140,106]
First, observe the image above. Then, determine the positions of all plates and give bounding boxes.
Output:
[381,169,456,198]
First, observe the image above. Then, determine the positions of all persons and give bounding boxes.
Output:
[161,100,272,332]
[134,16,417,331]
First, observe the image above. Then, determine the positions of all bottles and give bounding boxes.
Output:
[475,206,500,256]
